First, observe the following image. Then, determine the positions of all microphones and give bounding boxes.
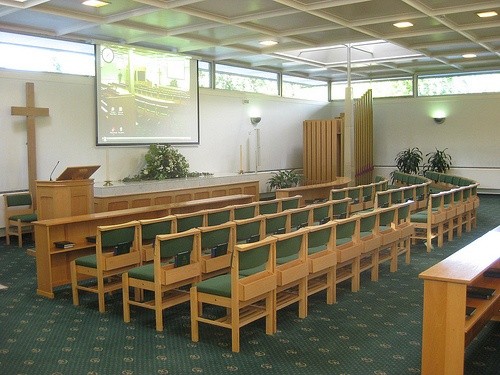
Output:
[49,160,59,180]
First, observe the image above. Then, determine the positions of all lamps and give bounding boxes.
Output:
[249,106,261,126]
[432,110,447,124]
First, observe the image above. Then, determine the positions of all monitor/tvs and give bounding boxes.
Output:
[55,165,101,181]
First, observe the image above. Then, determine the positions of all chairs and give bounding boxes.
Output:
[2,191,37,247]
[67,167,477,356]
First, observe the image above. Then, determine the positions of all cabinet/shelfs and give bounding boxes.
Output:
[417,223,500,375]
